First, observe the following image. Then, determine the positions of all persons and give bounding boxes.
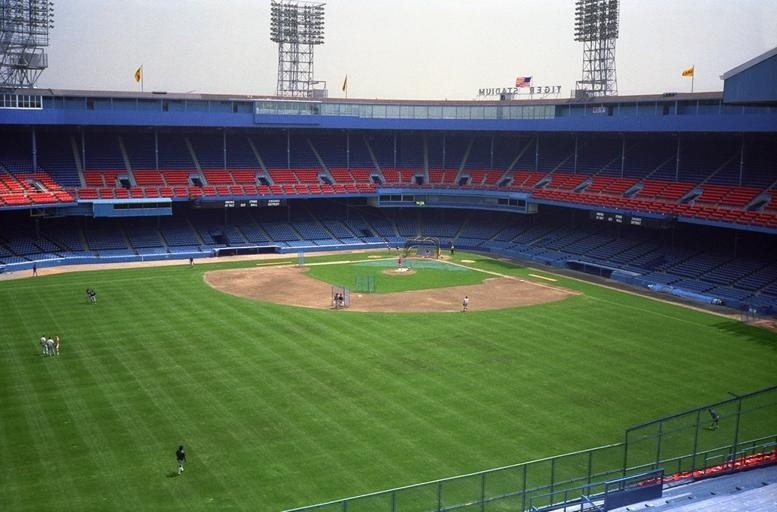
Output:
[398,255,402,268]
[40,335,60,357]
[396,245,400,251]
[175,445,187,476]
[450,243,455,255]
[190,256,193,266]
[708,406,720,431]
[462,296,470,312]
[86,288,97,305]
[33,263,38,277]
[333,293,344,310]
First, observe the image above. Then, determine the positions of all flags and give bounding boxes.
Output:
[682,67,694,76]
[134,66,140,82]
[343,76,346,91]
[516,77,531,88]
